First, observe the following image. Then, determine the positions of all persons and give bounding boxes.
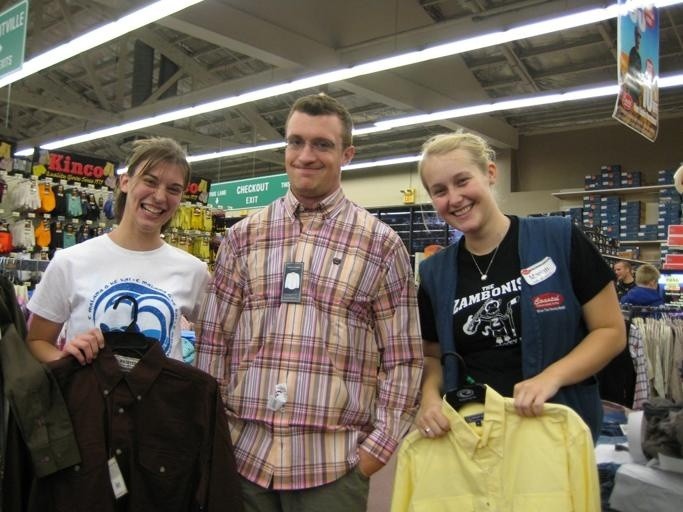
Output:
[190,92,423,512]
[621,263,666,321]
[416,125,628,447]
[25,135,213,370]
[611,260,635,302]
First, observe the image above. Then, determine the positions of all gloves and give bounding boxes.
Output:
[0,181,116,253]
[166,203,227,259]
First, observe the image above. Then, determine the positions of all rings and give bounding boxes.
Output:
[425,428,431,433]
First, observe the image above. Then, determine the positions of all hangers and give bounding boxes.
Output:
[425,352,519,424]
[84,294,168,380]
[620,302,683,330]
[0,256,39,292]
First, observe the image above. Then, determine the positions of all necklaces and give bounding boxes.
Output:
[467,240,502,281]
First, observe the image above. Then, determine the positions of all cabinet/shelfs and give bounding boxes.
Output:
[363,204,448,268]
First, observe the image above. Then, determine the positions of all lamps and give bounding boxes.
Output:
[0,0,682,174]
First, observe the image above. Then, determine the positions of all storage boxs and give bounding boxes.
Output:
[528,184,683,274]
